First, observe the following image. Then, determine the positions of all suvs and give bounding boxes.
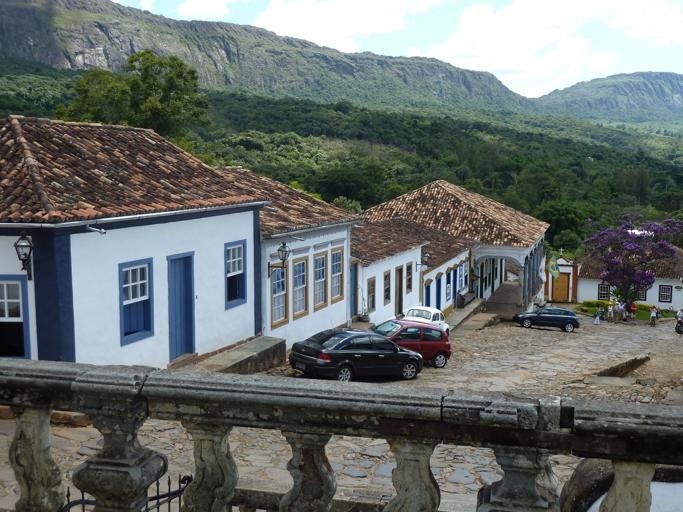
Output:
[402,305,452,340]
[513,307,582,334]
[357,318,454,372]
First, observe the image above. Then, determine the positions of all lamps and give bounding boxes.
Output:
[268,241,291,278]
[11,230,36,281]
[415,252,430,272]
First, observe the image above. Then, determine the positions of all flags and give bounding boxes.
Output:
[536,256,547,284]
[545,255,560,279]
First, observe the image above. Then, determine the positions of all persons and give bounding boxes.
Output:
[606,302,614,323]
[649,306,657,327]
[592,304,601,325]
[614,298,639,322]
[676,308,683,323]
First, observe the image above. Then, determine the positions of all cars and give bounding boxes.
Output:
[288,324,426,385]
